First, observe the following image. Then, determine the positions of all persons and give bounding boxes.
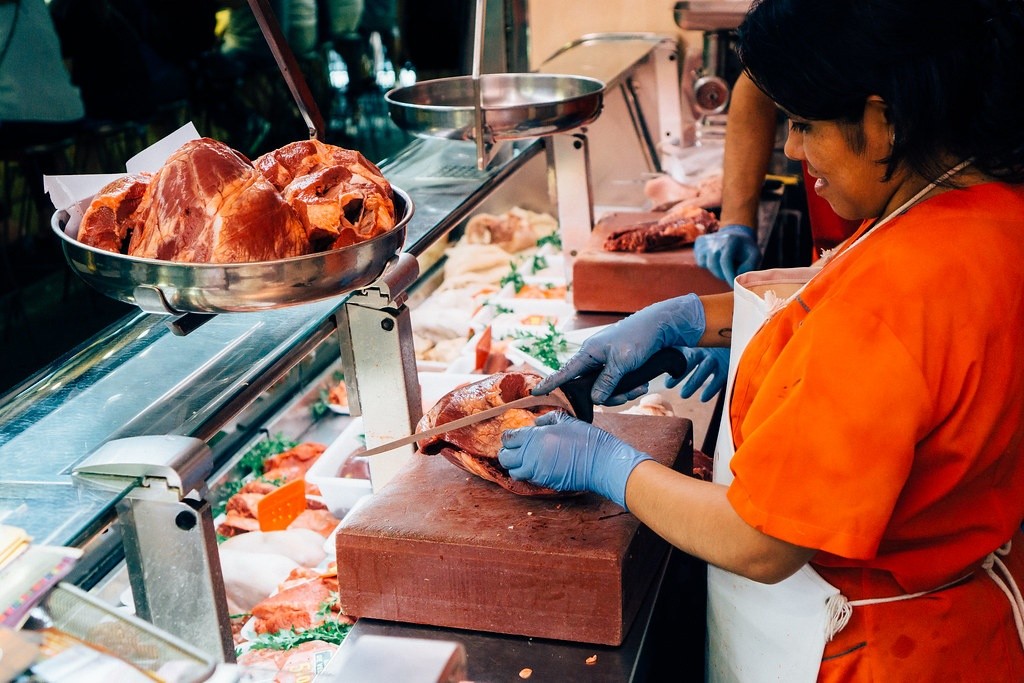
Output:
[499,0,1024,683]
[0,0,400,164]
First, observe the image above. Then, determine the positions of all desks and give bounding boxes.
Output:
[303,146,816,683]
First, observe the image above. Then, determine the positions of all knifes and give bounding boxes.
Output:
[358,347,689,457]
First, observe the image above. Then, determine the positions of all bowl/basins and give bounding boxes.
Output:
[49,182,414,314]
[384,74,607,139]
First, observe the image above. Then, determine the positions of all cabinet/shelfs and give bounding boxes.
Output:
[0,29,687,683]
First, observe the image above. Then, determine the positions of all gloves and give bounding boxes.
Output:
[664,343,731,402]
[694,225,761,290]
[496,409,659,513]
[532,291,707,407]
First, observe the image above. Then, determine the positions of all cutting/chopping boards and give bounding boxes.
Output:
[334,411,694,645]
[573,210,732,313]
